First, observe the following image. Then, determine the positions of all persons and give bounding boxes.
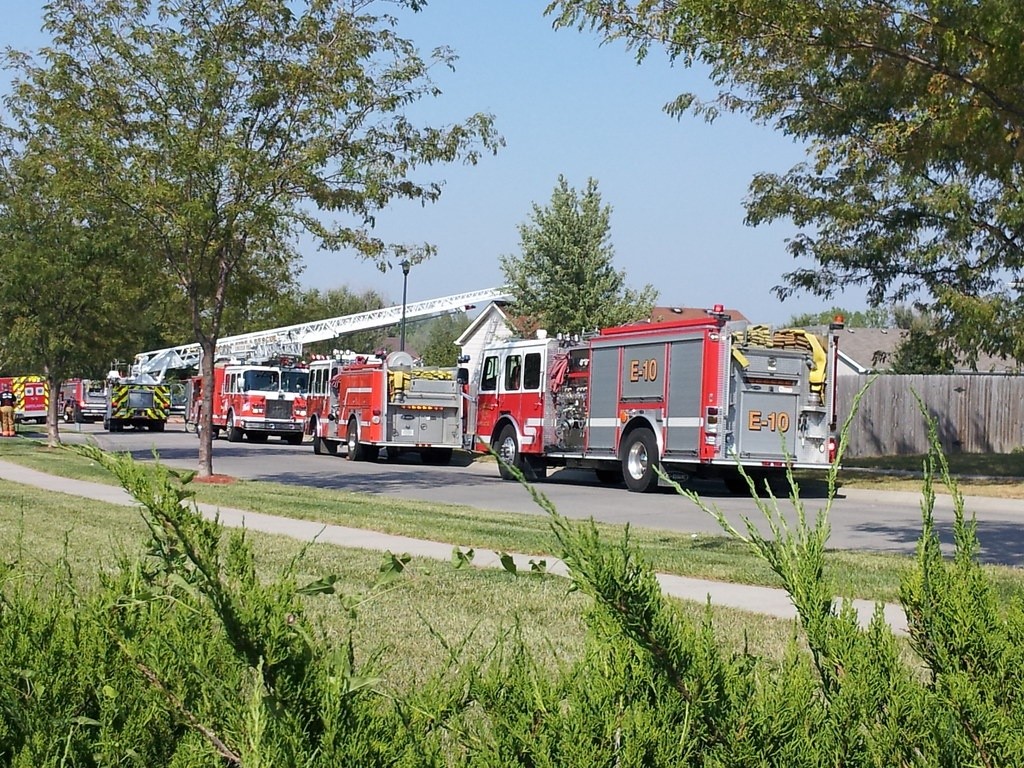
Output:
[0,383,16,437]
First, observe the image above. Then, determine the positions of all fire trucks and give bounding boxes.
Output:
[103,276,526,464]
[0,354,107,434]
[467,305,847,495]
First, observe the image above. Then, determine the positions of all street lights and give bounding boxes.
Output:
[398,259,413,350]
